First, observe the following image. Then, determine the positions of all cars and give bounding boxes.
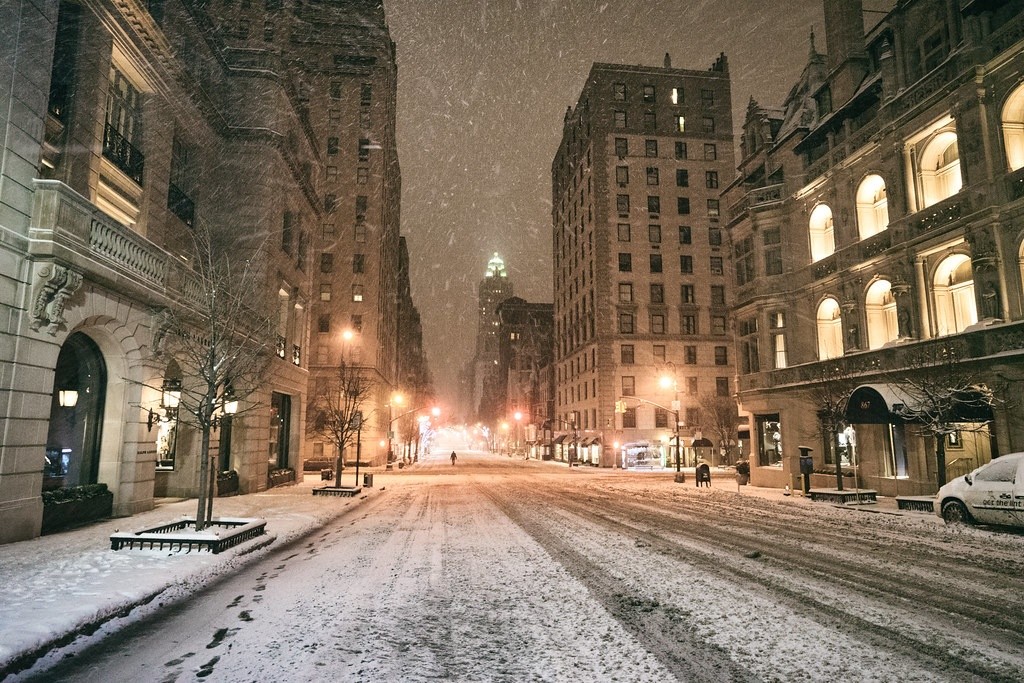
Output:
[933,451,1024,533]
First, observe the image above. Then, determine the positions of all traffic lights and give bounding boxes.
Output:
[616,401,626,414]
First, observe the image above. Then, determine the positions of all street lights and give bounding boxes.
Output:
[615,360,686,483]
[333,328,354,487]
[513,410,578,461]
[386,390,441,464]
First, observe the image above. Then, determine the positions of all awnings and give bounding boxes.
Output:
[553,435,599,446]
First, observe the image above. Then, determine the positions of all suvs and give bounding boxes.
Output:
[304,456,345,471]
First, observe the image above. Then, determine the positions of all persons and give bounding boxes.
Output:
[450,451,456,465]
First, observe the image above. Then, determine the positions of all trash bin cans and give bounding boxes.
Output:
[696,463,712,488]
[363,471,375,487]
